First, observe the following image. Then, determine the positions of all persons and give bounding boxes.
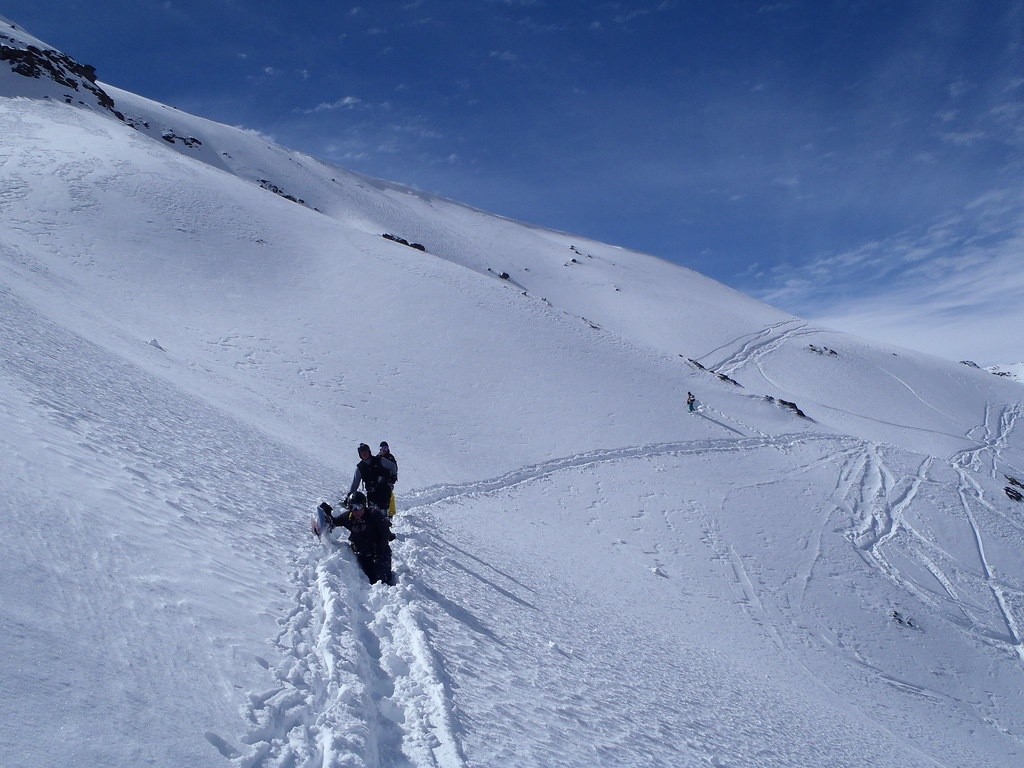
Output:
[340,439,398,537]
[323,491,402,590]
[372,441,402,521]
[686,391,696,414]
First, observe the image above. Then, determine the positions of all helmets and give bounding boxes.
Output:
[358,443,369,452]
[344,492,366,507]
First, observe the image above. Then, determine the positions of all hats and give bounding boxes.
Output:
[379,442,388,447]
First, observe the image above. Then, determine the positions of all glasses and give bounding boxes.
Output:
[379,447,388,450]
[348,504,365,511]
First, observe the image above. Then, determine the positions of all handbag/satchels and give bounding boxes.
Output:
[310,507,341,540]
